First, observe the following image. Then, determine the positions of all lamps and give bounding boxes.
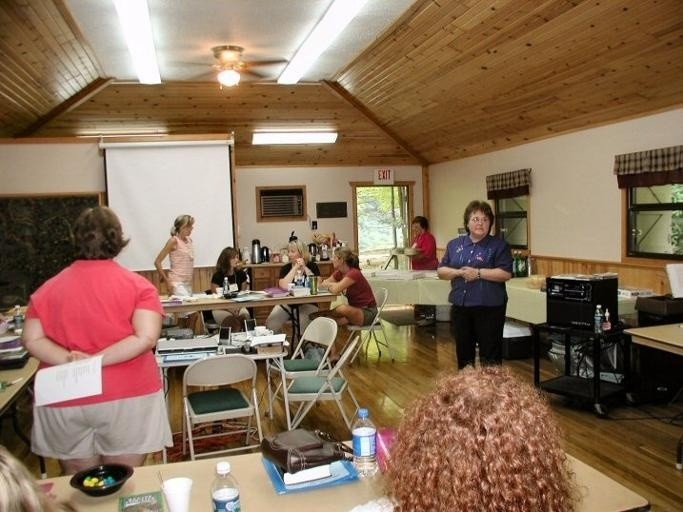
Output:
[250,130,339,146]
[217,71,242,91]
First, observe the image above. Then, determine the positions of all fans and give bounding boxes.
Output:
[172,45,290,82]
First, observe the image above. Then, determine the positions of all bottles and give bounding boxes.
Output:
[594,305,603,334]
[309,243,316,261]
[603,308,611,332]
[296,270,302,286]
[209,460,241,512]
[351,407,379,480]
[222,277,229,296]
[310,273,318,296]
[13,305,23,334]
[305,273,310,287]
[251,238,261,264]
[510,248,532,277]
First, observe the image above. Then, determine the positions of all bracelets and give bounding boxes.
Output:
[477,268,483,280]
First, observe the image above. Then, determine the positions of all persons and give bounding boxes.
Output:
[21,205,175,473]
[308,246,376,365]
[208,248,248,334]
[380,364,580,512]
[436,200,513,371]
[264,240,318,341]
[0,447,76,511]
[153,215,199,334]
[390,216,436,321]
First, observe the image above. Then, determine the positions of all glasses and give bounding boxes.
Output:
[318,432,352,454]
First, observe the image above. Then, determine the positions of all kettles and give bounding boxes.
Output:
[261,246,271,262]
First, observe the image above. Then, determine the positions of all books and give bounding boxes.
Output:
[273,461,333,489]
[158,284,313,306]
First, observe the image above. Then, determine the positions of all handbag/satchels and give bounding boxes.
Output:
[260,428,346,475]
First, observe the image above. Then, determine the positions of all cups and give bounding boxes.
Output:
[254,325,266,337]
[161,477,193,511]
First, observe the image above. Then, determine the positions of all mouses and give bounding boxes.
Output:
[241,344,253,353]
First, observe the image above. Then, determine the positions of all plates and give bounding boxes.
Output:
[69,462,134,497]
[223,293,238,298]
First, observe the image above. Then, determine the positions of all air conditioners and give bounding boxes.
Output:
[261,194,303,217]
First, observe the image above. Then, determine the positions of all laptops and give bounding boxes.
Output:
[156,338,218,355]
